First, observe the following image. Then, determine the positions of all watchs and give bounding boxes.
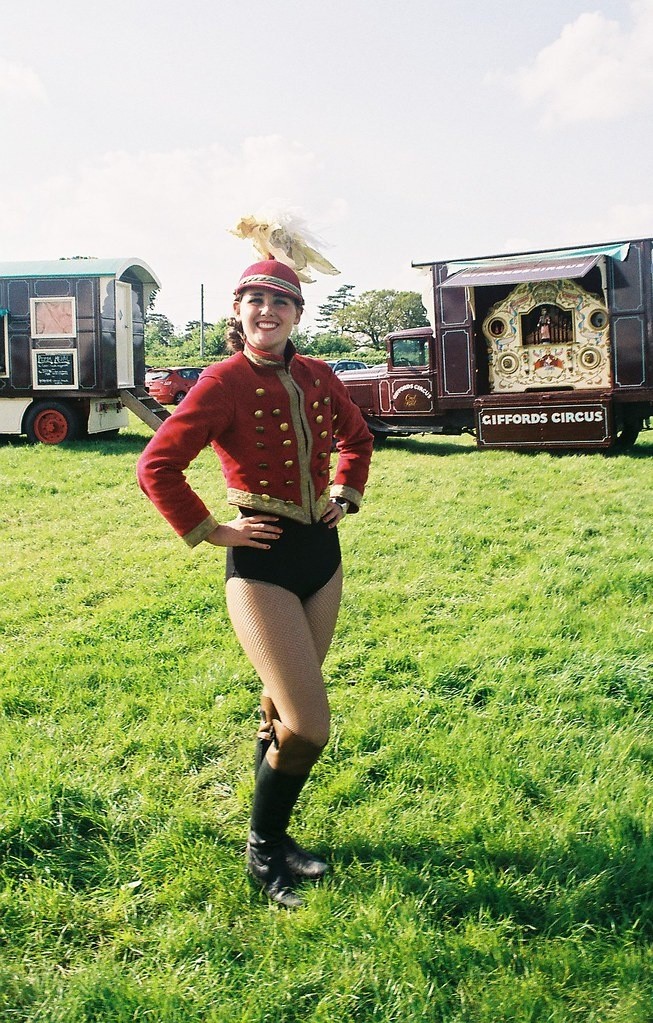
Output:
[329,497,348,517]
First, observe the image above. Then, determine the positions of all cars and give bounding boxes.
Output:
[142,356,366,401]
[329,242,653,459]
[1,259,175,444]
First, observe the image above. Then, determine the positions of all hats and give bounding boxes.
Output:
[226,213,342,305]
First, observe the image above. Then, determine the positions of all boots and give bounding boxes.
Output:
[245,718,308,910]
[256,695,334,880]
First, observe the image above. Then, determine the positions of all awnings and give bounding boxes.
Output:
[436,255,601,288]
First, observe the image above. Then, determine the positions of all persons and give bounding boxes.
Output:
[537,309,551,344]
[137,260,373,908]
